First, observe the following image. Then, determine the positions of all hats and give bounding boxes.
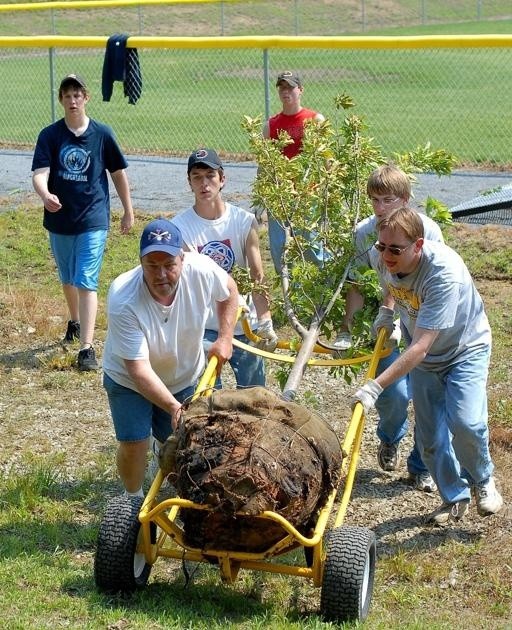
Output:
[59,73,87,90]
[188,147,225,171]
[138,215,184,260]
[276,71,303,89]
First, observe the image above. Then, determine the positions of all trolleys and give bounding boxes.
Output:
[93,291,397,626]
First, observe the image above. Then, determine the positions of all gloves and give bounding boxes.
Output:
[372,305,397,339]
[329,331,354,360]
[253,317,279,353]
[349,377,385,417]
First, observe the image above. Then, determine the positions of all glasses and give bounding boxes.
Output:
[374,238,417,256]
[365,195,402,206]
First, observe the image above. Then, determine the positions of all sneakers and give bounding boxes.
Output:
[412,472,438,493]
[377,439,402,472]
[77,343,100,371]
[63,320,81,341]
[473,475,504,517]
[424,502,469,527]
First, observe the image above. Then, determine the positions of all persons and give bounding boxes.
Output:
[101,216,239,498]
[29,72,134,370]
[167,146,279,390]
[250,71,330,276]
[350,204,505,526]
[331,166,447,493]
